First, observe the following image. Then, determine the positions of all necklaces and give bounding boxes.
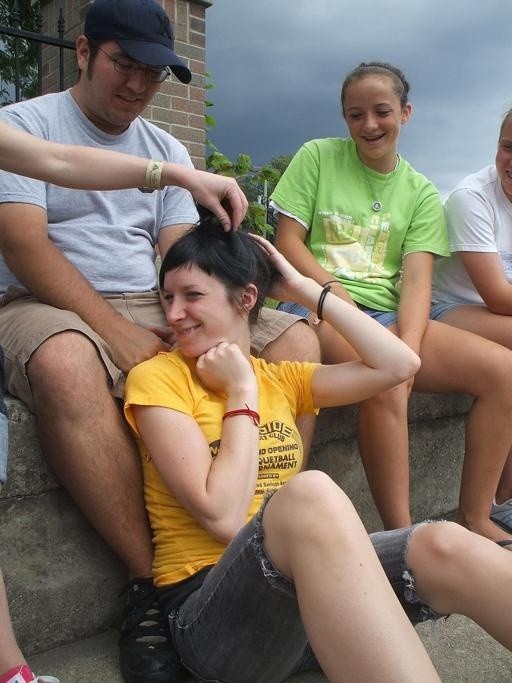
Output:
[358,154,399,211]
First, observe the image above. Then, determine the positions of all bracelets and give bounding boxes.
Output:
[317,286,331,320]
[144,159,165,190]
[222,409,263,427]
[322,280,343,287]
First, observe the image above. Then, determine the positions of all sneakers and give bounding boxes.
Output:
[118,576,182,682]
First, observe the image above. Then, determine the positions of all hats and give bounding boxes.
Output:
[83,0,194,86]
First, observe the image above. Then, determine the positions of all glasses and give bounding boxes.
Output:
[96,47,170,84]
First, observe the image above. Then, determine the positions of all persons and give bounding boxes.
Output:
[0,0,321,683]
[124,224,512,683]
[0,120,249,683]
[269,61,512,552]
[430,108,512,351]
